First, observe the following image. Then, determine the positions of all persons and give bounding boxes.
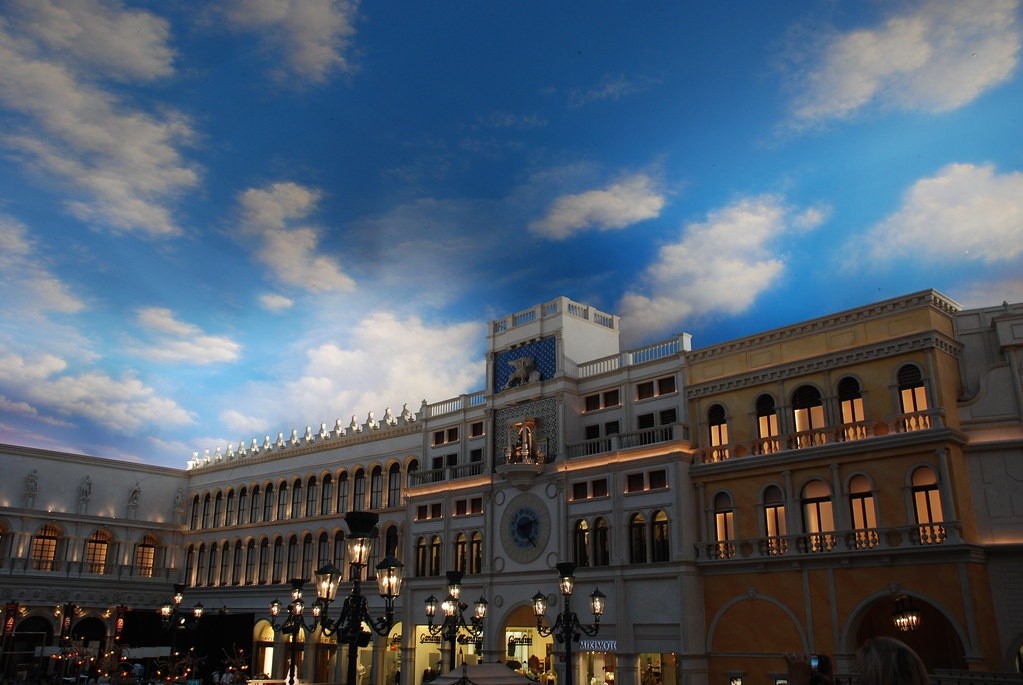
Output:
[211,667,249,685]
[394,667,401,685]
[422,666,437,684]
[357,663,366,685]
[129,481,141,504]
[26,468,39,493]
[783,637,930,685]
[527,655,540,670]
[79,474,92,500]
[174,487,184,508]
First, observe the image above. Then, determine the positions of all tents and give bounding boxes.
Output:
[428,662,537,685]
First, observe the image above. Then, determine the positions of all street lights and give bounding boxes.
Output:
[314,533,405,685]
[159,588,204,685]
[424,571,488,673]
[531,561,607,685]
[270,586,323,685]
